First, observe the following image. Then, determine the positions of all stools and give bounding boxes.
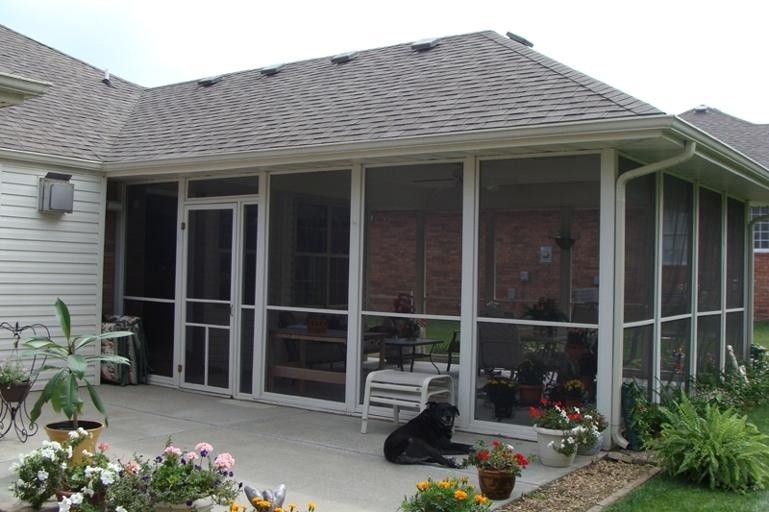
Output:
[360,369,456,434]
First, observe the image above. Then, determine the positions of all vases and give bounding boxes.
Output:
[534,423,581,469]
[167,495,212,512]
[494,397,513,419]
[478,467,515,499]
[52,489,82,509]
[564,395,581,407]
[578,429,605,456]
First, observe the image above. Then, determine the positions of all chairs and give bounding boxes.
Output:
[478,315,525,380]
[366,325,393,366]
[447,330,460,372]
[567,301,600,350]
[277,312,333,388]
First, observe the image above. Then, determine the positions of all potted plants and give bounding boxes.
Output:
[515,358,552,406]
[0,363,32,405]
[564,331,587,361]
[21,297,136,469]
[305,288,328,334]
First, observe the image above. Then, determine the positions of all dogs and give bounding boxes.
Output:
[384,401,476,469]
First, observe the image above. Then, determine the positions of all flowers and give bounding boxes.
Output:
[393,294,428,341]
[110,436,244,512]
[548,400,609,456]
[479,378,514,402]
[10,428,119,511]
[529,399,585,433]
[553,376,591,400]
[461,438,528,477]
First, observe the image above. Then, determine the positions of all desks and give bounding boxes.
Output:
[268,324,386,405]
[382,338,445,374]
[520,334,572,375]
